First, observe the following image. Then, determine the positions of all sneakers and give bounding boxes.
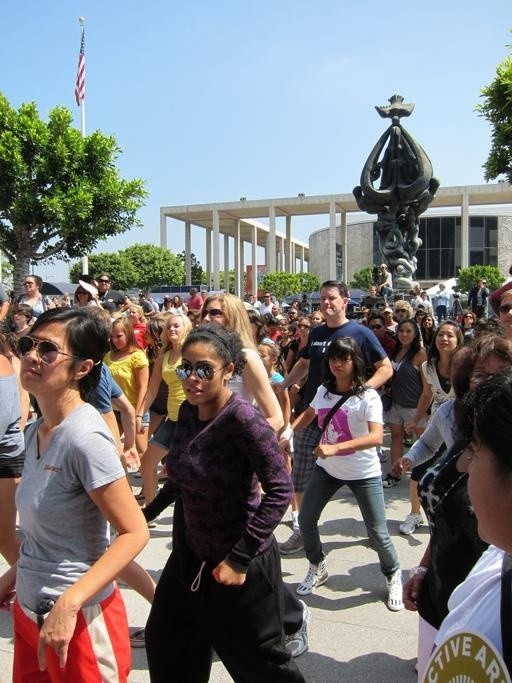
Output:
[383,472,401,487]
[399,512,424,534]
[284,600,312,658]
[377,448,388,463]
[384,570,405,611]
[296,560,329,596]
[130,628,146,648]
[280,529,304,554]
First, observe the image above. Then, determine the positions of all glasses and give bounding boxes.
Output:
[395,309,406,313]
[23,282,33,286]
[462,437,482,462]
[202,309,224,317]
[18,335,86,365]
[288,312,297,315]
[99,278,109,283]
[498,305,511,313]
[263,295,270,298]
[174,365,225,382]
[76,291,88,295]
[369,324,382,330]
[298,324,310,329]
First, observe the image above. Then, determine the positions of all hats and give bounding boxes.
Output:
[138,292,145,296]
[490,281,512,316]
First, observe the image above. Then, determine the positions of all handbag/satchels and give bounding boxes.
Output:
[381,392,392,412]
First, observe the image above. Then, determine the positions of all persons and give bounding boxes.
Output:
[277,334,405,612]
[144,323,306,682]
[136,314,192,529]
[1,307,148,683]
[383,320,426,489]
[269,280,393,554]
[100,317,150,500]
[200,293,310,659]
[403,336,512,683]
[78,361,157,648]
[362,265,512,362]
[1,333,25,564]
[388,397,456,479]
[467,369,512,678]
[399,321,465,536]
[242,293,311,373]
[0,276,204,358]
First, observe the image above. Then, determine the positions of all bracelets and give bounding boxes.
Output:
[410,565,427,576]
